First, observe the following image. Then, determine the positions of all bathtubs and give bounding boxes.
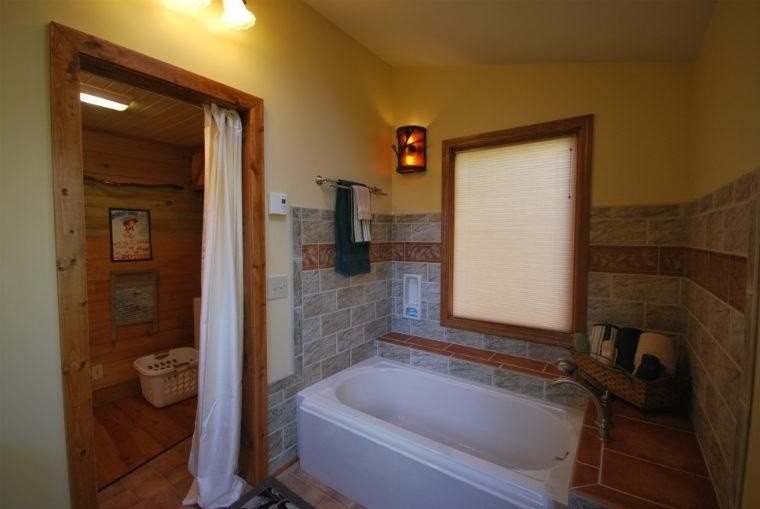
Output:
[296,358,584,508]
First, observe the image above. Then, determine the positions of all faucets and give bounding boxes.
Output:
[551,376,607,441]
[555,357,611,414]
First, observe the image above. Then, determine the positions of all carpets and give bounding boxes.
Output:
[227,476,318,509]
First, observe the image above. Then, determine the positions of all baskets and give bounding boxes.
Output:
[570,348,690,409]
[133,347,198,409]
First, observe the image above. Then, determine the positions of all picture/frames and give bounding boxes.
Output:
[109,208,153,263]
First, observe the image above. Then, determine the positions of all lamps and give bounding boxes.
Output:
[221,0,257,33]
[78,82,134,112]
[392,126,427,174]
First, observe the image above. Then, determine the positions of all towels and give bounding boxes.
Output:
[336,179,371,276]
[589,323,677,377]
[350,185,372,244]
